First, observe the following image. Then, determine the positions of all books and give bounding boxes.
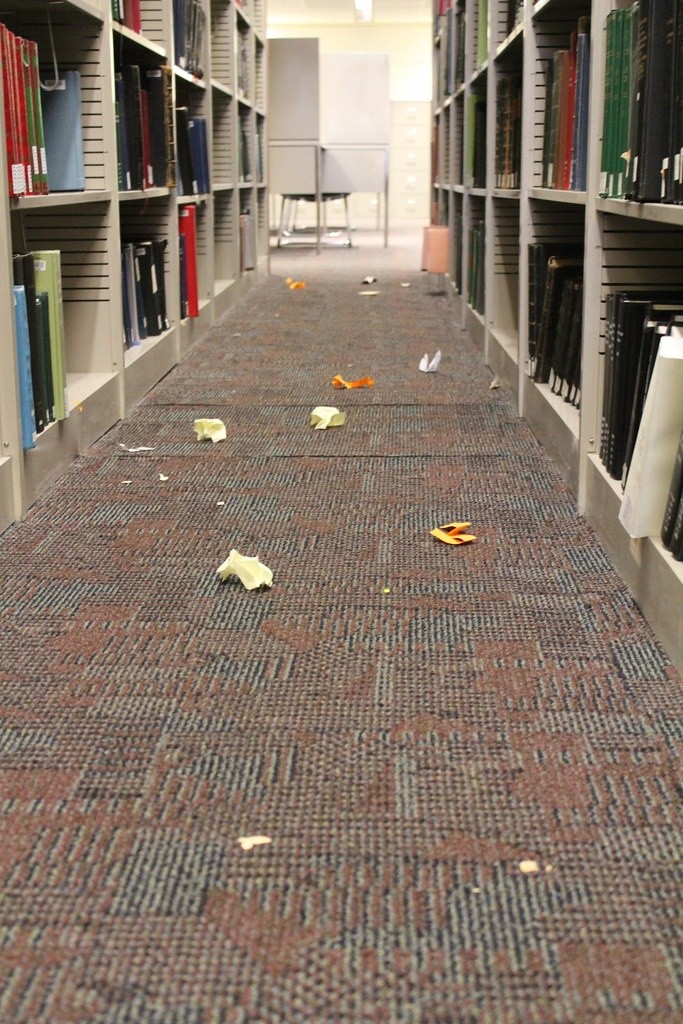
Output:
[173,0,206,79]
[237,29,248,98]
[240,209,254,274]
[495,72,522,189]
[421,0,489,315]
[527,242,584,410]
[114,63,178,191]
[0,21,86,198]
[496,0,523,49]
[120,235,170,351]
[235,0,245,10]
[542,16,591,191]
[600,291,683,562]
[598,0,683,206]
[13,249,69,450]
[178,204,199,320]
[238,114,253,182]
[110,0,142,35]
[255,133,262,183]
[176,107,211,197]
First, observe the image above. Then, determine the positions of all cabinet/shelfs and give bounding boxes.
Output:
[0,0,683,680]
